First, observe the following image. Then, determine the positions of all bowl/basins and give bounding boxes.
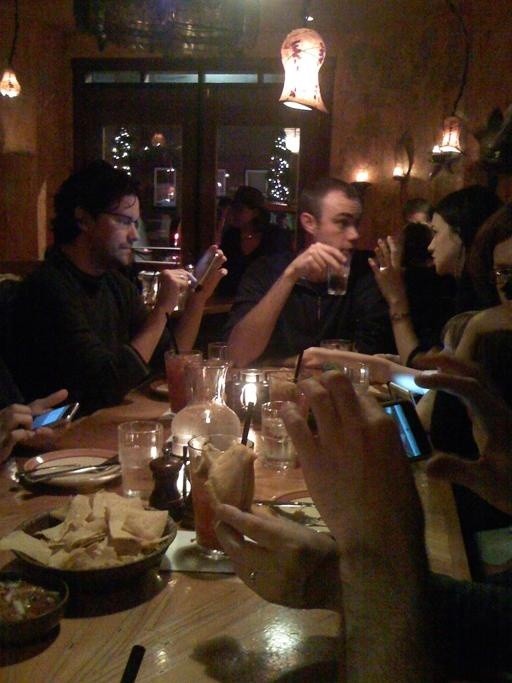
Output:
[11,504,177,584]
[1,568,70,640]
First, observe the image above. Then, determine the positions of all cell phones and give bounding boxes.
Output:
[389,382,416,410]
[18,402,80,432]
[190,253,219,293]
[312,397,434,465]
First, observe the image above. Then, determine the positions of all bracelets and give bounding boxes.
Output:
[390,310,412,321]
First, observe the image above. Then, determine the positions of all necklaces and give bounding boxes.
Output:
[240,231,259,238]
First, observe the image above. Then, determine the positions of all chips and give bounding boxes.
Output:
[0,491,168,568]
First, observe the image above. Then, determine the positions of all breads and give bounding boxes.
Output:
[203,443,258,511]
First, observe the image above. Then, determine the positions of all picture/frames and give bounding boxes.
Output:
[243,167,268,197]
[152,165,176,207]
[215,167,229,198]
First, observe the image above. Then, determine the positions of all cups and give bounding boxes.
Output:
[187,433,254,560]
[326,250,353,293]
[117,418,161,497]
[163,340,370,497]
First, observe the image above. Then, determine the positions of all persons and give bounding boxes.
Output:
[209,498,512,665]
[283,345,511,680]
[367,183,504,368]
[401,197,435,226]
[215,185,294,296]
[452,196,512,362]
[0,388,70,463]
[299,310,478,435]
[0,157,229,419]
[222,175,396,370]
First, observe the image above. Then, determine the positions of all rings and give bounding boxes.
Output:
[247,567,258,583]
[380,262,393,272]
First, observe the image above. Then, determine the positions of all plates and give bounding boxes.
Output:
[269,489,335,537]
[22,446,121,487]
[149,378,170,396]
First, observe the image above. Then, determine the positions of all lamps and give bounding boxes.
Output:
[0,0,23,97]
[432,0,470,152]
[390,164,408,200]
[282,126,300,155]
[349,168,372,197]
[273,0,329,116]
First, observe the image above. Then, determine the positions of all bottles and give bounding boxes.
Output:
[183,455,209,530]
[149,444,185,516]
